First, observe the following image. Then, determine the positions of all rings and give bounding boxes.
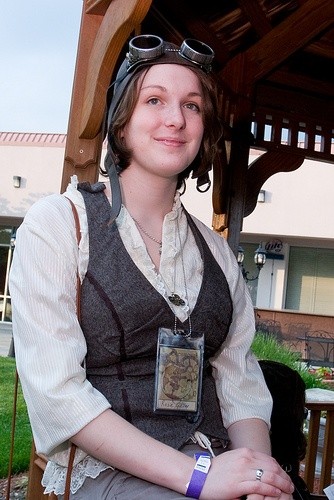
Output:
[256,469,264,481]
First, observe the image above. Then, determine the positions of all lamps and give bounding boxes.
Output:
[13,176,22,189]
[237,242,268,284]
[257,190,265,202]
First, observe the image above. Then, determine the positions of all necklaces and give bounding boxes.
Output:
[129,212,163,255]
[135,218,186,306]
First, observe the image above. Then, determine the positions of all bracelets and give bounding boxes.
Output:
[185,452,211,499]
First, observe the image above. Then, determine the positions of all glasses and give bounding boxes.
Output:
[299,407,309,420]
[103,34,215,136]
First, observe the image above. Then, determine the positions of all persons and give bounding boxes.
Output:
[9,34,296,500]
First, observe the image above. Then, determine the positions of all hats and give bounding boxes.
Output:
[107,40,214,169]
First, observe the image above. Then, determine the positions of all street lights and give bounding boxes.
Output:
[2,233,17,321]
